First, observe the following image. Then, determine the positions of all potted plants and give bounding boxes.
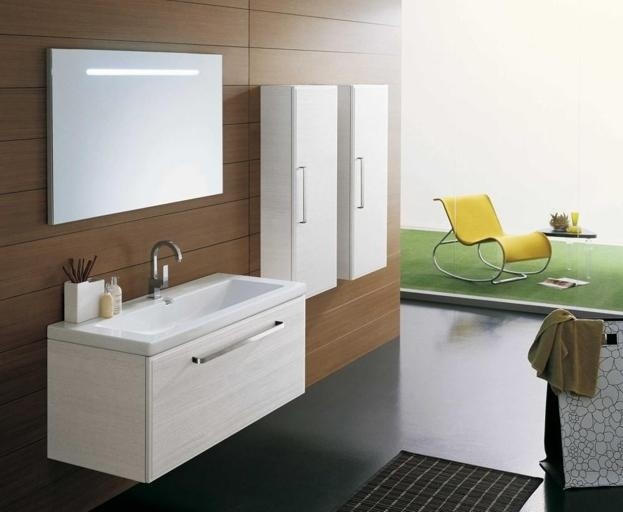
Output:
[547,208,569,232]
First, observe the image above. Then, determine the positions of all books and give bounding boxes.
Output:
[538,276,591,289]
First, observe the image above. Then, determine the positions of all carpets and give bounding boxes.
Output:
[335,451,543,512]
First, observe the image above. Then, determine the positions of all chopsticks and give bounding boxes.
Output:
[62,254,98,282]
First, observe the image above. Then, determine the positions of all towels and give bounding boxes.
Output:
[526,308,603,400]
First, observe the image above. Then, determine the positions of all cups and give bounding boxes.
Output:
[571,211,580,227]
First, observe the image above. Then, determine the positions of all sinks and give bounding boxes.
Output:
[94,273,285,337]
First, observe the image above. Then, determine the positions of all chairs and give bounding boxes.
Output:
[430,194,552,286]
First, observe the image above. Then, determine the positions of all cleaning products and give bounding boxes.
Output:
[100,288,114,318]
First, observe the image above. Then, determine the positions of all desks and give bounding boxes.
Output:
[534,226,597,280]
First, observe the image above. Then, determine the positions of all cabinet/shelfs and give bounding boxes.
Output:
[337,83,388,281]
[259,82,338,298]
[46,296,308,485]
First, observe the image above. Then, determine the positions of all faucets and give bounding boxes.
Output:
[148,240,183,299]
